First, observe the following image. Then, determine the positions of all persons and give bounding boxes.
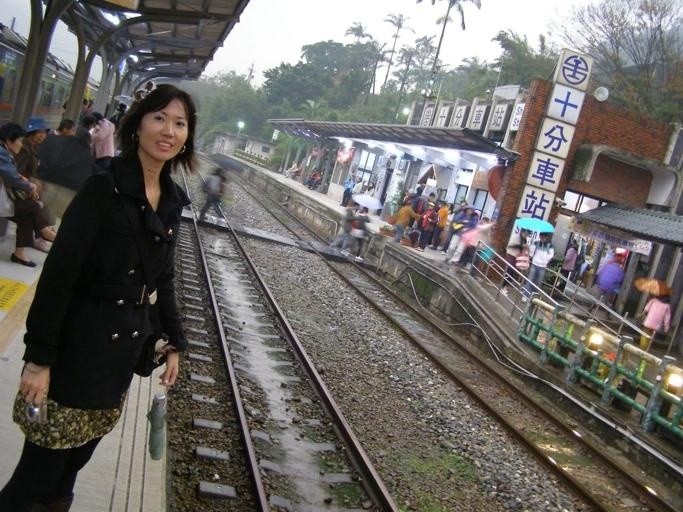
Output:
[0,121,58,268]
[555,238,578,294]
[328,171,490,271]
[198,165,226,220]
[636,294,671,352]
[585,252,625,324]
[0,82,197,512]
[45,92,126,176]
[12,116,57,253]
[520,232,554,302]
[283,160,324,190]
[499,228,532,297]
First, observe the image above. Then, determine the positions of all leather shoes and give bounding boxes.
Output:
[11,253,36,266]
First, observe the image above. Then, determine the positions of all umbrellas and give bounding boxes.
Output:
[146,393,167,460]
[631,277,675,298]
[512,216,556,247]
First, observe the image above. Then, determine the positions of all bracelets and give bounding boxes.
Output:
[23,363,50,374]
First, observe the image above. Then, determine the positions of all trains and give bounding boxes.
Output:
[0,24,111,132]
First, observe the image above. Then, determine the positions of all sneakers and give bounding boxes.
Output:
[33,234,54,252]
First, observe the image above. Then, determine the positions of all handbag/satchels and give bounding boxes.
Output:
[453,223,464,229]
[516,256,530,270]
[134,289,175,377]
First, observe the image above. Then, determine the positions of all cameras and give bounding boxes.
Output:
[23,389,49,427]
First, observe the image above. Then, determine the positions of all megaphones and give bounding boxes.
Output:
[594,86,609,101]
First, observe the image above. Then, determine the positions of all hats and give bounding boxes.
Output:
[25,119,50,133]
